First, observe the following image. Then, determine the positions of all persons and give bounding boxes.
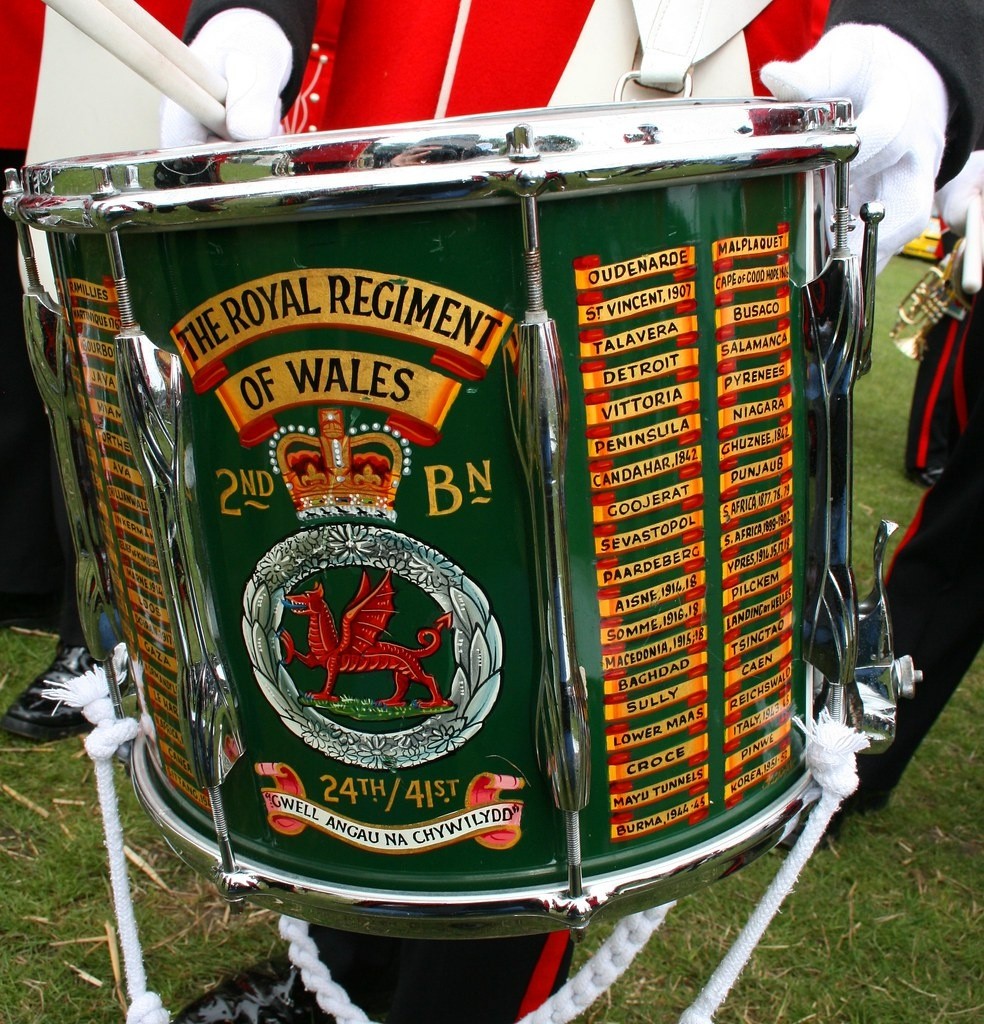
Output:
[0,1,984,851]
[160,0,984,1024]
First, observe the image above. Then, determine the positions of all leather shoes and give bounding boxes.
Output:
[174,944,402,1024]
[2,645,129,741]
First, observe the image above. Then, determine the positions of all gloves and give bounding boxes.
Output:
[161,6,293,149]
[935,147,983,295]
[759,23,949,277]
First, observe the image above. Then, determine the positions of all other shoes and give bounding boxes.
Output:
[779,781,873,852]
[905,465,944,488]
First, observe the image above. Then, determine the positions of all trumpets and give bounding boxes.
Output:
[890,240,967,362]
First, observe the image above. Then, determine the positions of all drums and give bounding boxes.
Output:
[6,92,914,943]
